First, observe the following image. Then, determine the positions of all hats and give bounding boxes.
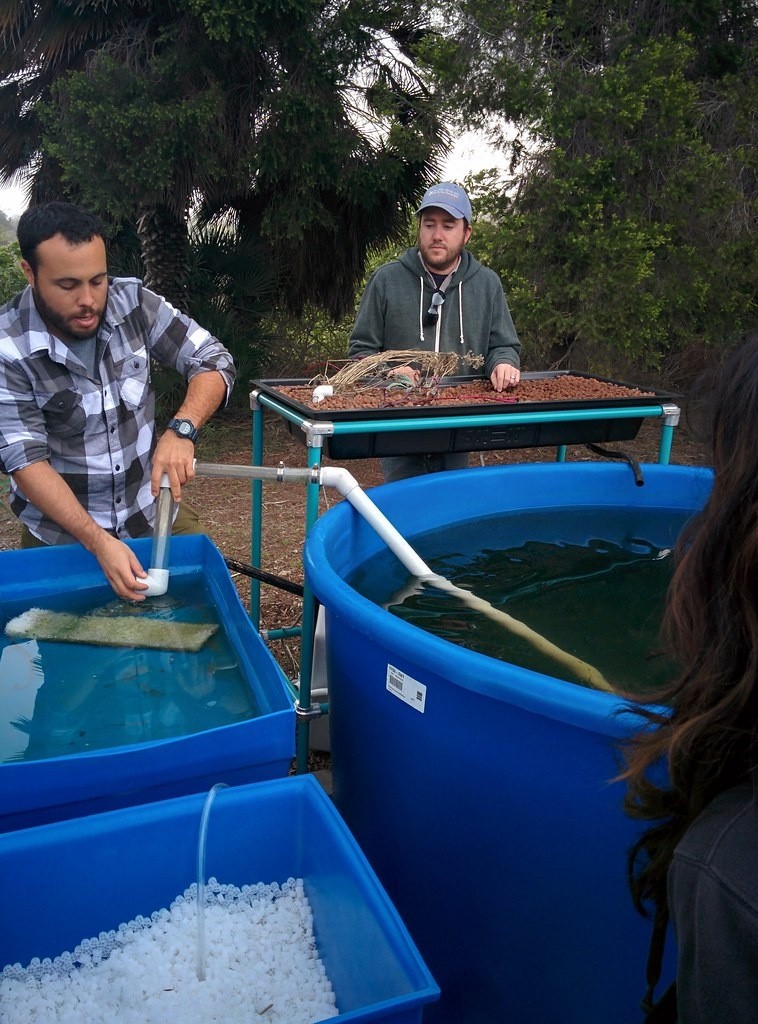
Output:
[414,183,470,226]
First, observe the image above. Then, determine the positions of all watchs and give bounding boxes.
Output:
[167,418,199,444]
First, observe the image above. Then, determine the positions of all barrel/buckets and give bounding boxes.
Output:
[302,463,715,1024]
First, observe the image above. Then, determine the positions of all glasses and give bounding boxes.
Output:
[424,287,446,328]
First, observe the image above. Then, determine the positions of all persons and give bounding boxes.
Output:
[596,334,758,1024]
[1,201,235,600]
[348,182,521,484]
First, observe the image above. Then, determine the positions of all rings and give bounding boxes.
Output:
[511,375,516,380]
